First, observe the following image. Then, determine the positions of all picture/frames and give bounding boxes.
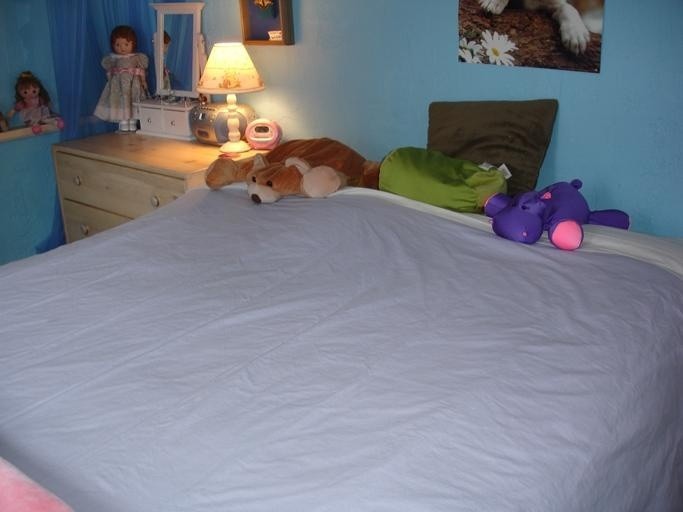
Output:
[238,0,294,46]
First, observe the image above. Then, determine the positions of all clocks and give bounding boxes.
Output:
[246,118,283,150]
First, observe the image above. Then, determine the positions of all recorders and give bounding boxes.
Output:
[190,103,256,146]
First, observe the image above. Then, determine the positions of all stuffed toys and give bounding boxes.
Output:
[205,137,379,203]
[484,179,630,250]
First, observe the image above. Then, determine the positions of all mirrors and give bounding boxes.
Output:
[146,2,208,100]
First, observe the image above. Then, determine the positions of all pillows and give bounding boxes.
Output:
[425,98,558,198]
[376,147,512,214]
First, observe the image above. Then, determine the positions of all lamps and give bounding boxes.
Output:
[194,41,265,154]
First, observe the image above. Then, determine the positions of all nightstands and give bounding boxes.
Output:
[51,128,271,243]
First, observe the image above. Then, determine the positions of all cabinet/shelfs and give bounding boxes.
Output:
[131,97,204,141]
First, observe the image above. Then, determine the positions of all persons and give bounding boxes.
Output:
[93,25,149,131]
[8,71,63,134]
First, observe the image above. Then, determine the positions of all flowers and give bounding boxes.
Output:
[457,28,519,65]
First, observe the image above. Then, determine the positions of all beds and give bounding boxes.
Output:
[0,182,682,512]
